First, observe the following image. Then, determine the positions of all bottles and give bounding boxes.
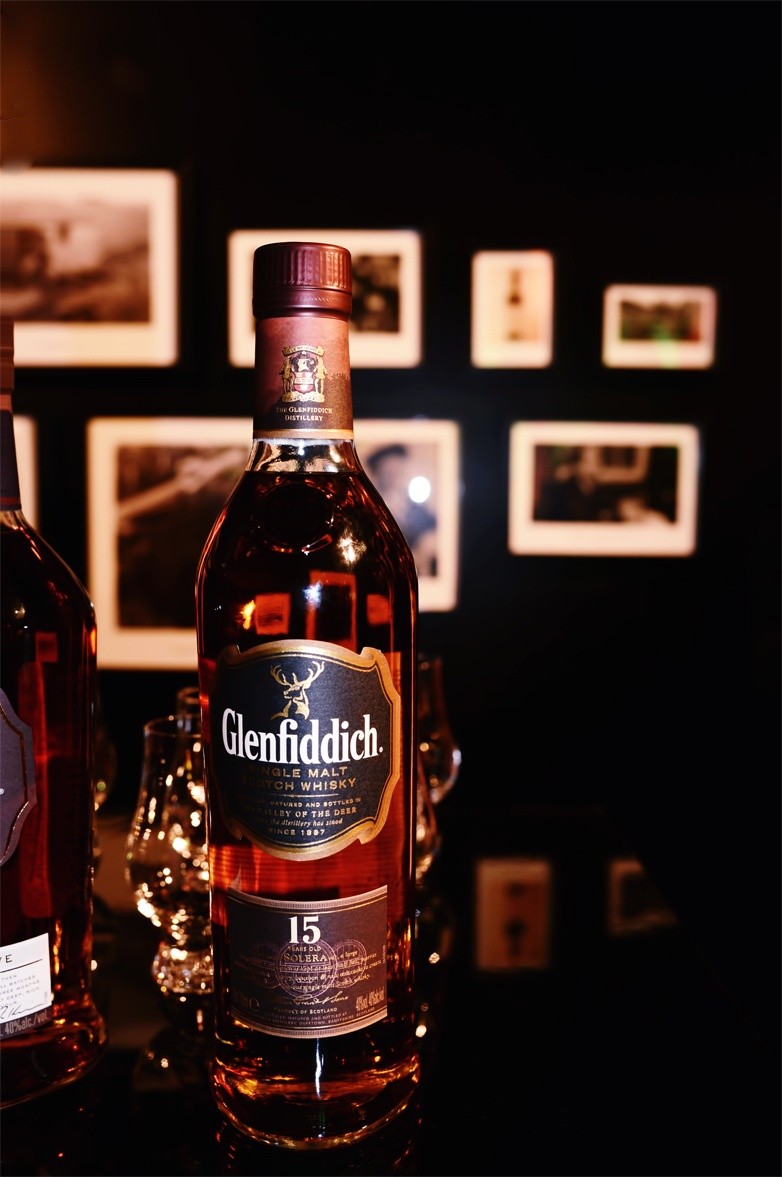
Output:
[0,315,104,1111]
[196,243,428,1149]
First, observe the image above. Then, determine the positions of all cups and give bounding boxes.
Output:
[124,653,461,995]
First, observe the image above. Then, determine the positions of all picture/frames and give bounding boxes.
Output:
[87,415,461,670]
[510,422,700,556]
[0,167,176,367]
[228,228,419,367]
[470,251,553,366]
[601,284,717,369]
